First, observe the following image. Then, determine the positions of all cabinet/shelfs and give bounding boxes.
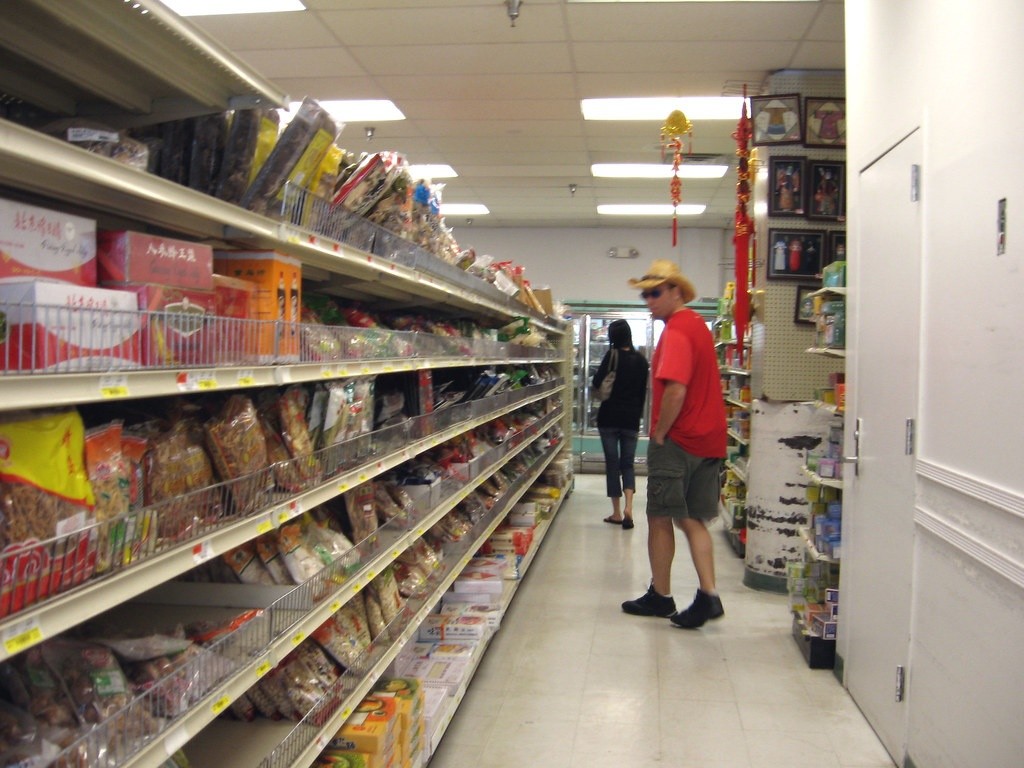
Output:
[0,0,574,768]
[803,287,846,669]
[709,289,753,558]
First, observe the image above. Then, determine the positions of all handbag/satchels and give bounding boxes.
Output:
[591,348,618,402]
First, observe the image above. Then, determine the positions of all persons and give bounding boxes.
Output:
[592,319,650,530]
[621,261,729,628]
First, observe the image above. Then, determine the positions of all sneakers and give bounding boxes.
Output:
[622,585,678,618]
[670,588,723,627]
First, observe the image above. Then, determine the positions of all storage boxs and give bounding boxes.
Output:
[307,558,507,768]
[0,197,302,374]
[451,457,479,479]
[786,261,846,640]
[401,477,441,507]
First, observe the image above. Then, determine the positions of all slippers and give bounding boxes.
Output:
[622,519,634,529]
[603,516,623,524]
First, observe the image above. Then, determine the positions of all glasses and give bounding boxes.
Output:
[641,285,676,299]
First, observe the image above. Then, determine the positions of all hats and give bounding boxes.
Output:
[628,259,696,303]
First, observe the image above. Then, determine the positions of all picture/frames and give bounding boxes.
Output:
[750,91,846,325]
